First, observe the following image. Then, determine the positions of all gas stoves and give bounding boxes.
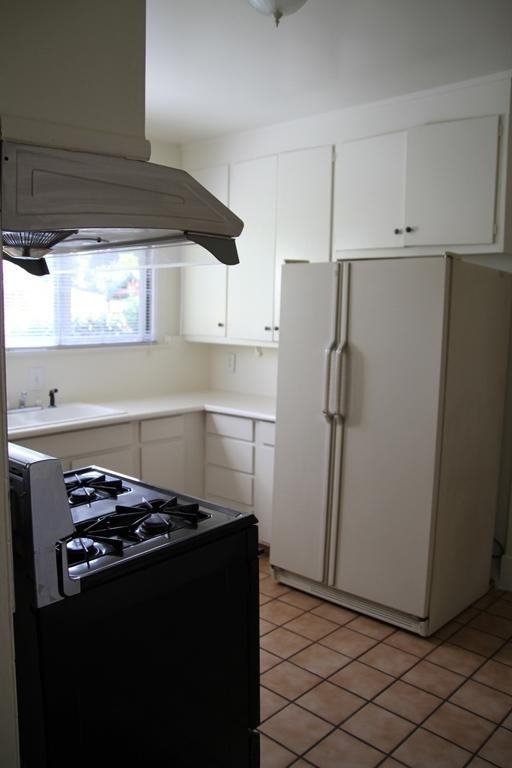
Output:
[62,464,259,581]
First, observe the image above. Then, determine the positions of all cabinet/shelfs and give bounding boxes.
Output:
[229,147,331,342]
[203,411,256,514]
[179,166,228,339]
[16,424,138,478]
[334,113,512,258]
[256,420,274,548]
[139,415,186,494]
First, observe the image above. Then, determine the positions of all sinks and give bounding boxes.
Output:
[7,401,129,430]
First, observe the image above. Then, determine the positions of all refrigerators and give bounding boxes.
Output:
[268,251,512,639]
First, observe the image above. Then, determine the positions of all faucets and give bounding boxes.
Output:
[18,392,29,411]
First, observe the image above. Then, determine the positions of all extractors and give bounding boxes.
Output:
[1,141,245,276]
